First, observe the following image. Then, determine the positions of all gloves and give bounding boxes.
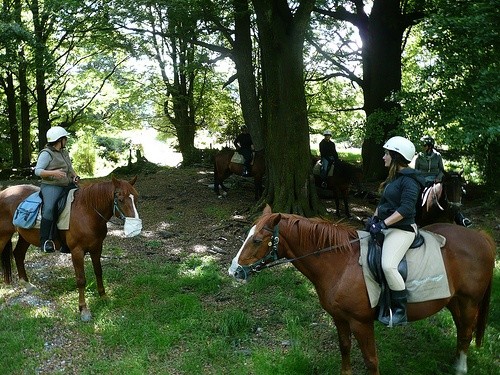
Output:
[370,220,389,234]
[366,216,378,230]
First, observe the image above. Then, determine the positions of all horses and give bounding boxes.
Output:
[214,146,265,203]
[0,174,142,321]
[312,158,368,218]
[229,202,497,375]
[414,168,464,227]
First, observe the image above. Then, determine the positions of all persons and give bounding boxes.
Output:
[415,135,471,226]
[35,126,79,253]
[319,130,336,188]
[234,125,256,177]
[366,136,425,326]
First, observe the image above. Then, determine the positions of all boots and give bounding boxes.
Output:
[40,218,53,252]
[378,289,408,326]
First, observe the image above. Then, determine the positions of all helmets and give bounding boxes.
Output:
[46,126,71,143]
[323,129,332,135]
[420,135,435,145]
[383,136,416,162]
[240,125,247,129]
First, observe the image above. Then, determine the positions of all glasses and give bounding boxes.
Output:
[60,137,67,141]
